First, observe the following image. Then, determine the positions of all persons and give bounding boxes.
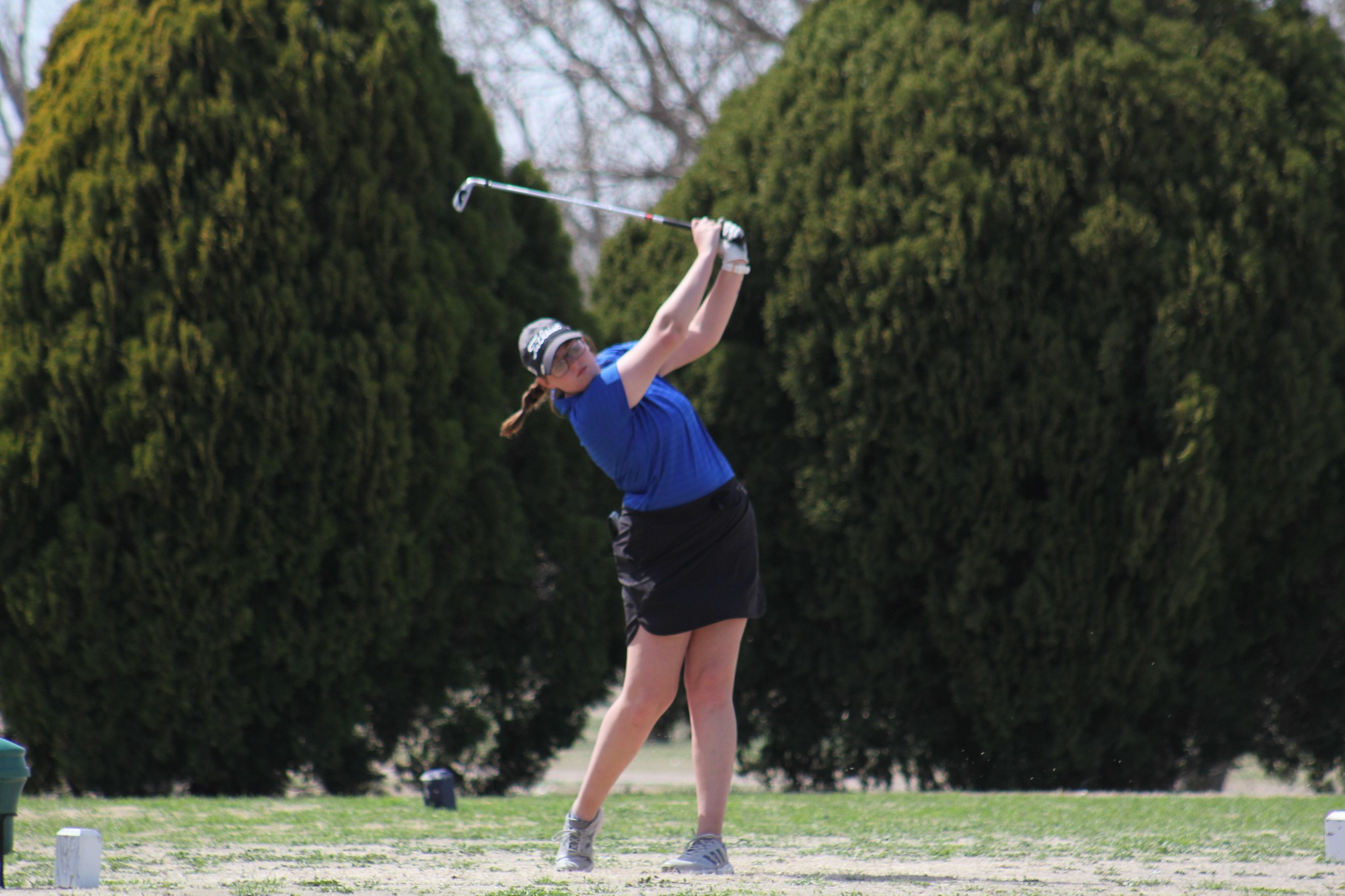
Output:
[500,217,761,876]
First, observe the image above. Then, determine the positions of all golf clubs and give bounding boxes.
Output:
[452,176,748,248]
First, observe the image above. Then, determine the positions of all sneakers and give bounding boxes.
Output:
[552,808,604,873]
[662,829,734,874]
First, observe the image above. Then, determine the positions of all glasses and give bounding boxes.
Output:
[550,338,585,378]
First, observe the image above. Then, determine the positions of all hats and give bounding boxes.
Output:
[518,318,583,377]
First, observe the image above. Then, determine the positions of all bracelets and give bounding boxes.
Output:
[722,263,750,275]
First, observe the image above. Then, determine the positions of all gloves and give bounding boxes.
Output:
[717,221,749,263]
